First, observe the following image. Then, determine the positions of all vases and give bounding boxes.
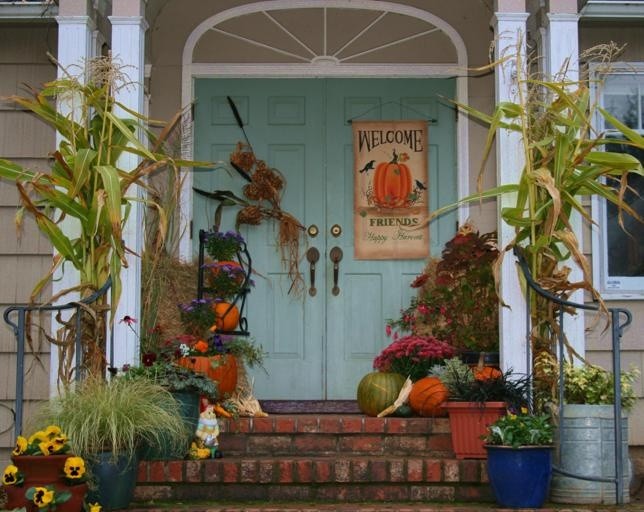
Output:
[10,456,66,484]
[141,393,197,460]
[458,349,499,365]
[5,485,86,512]
[485,444,555,509]
[214,302,239,331]
[174,354,237,402]
[209,261,241,286]
[555,405,630,505]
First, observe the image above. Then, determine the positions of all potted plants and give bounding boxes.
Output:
[40,375,194,512]
[413,365,546,460]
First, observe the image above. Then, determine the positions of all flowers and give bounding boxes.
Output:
[25,485,73,511]
[11,425,72,456]
[202,262,256,303]
[108,315,218,399]
[200,224,246,262]
[387,284,499,352]
[407,219,500,295]
[82,493,103,512]
[374,335,456,383]
[3,466,26,486]
[173,298,221,356]
[480,406,557,450]
[64,456,91,484]
[556,356,642,409]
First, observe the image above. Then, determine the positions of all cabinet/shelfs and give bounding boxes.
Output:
[198,229,252,336]
